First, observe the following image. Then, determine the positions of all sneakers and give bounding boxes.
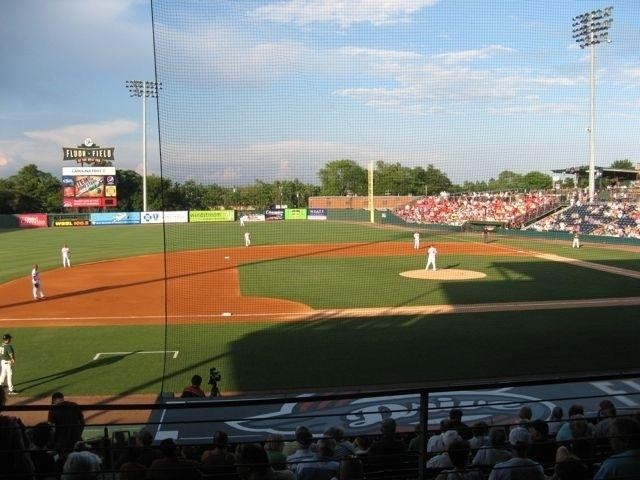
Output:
[8,391,19,395]
[34,297,40,301]
[41,297,48,301]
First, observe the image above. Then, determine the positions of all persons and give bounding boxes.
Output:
[31,263,46,300]
[180,374,206,398]
[424,243,437,272]
[239,215,245,227]
[392,183,639,251]
[0,383,640,479]
[244,231,252,248]
[60,244,73,269]
[0,333,19,395]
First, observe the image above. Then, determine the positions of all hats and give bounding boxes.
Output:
[510,428,532,445]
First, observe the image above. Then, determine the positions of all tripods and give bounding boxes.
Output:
[211,387,221,397]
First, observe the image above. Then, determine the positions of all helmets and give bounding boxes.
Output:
[3,333,13,339]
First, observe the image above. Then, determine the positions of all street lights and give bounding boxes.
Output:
[571,3,612,204]
[124,78,163,213]
